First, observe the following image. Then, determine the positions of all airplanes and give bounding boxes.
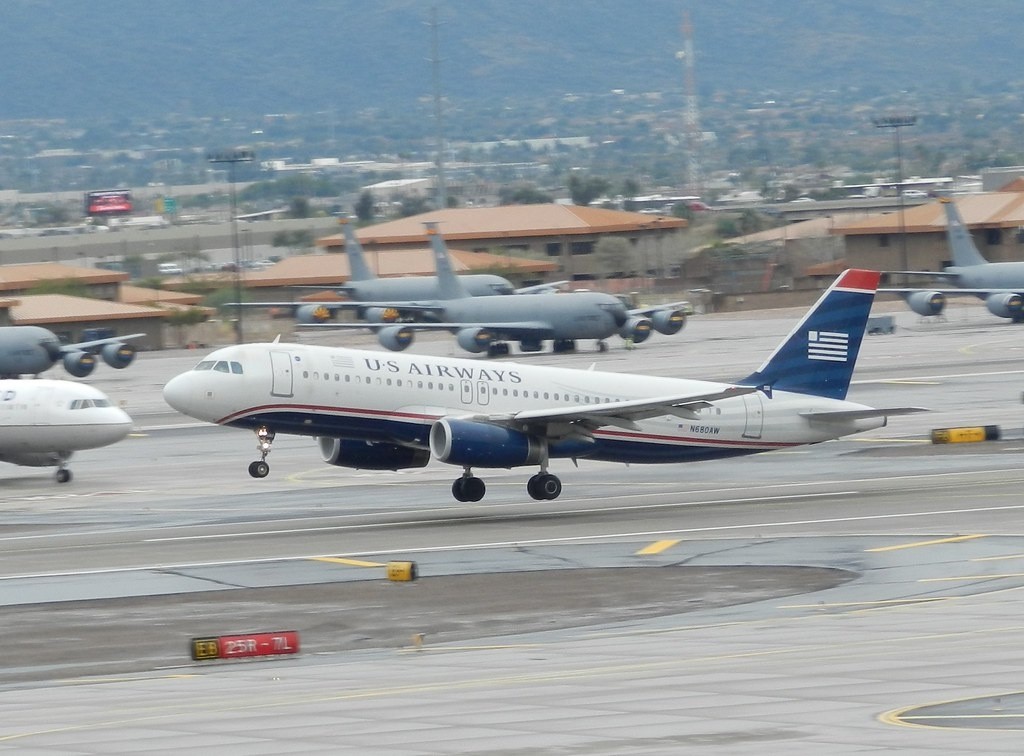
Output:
[294,221,689,353]
[162,268,929,502]
[0,378,134,483]
[223,211,570,335]
[876,202,1024,319]
[0,326,147,379]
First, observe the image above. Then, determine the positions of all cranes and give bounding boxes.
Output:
[683,10,700,197]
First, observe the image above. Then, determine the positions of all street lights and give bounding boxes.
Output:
[206,151,255,344]
[872,116,917,289]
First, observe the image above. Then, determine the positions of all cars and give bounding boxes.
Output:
[159,264,180,274]
[639,208,663,215]
[789,198,815,203]
[902,190,927,199]
[256,260,275,266]
[268,256,282,262]
